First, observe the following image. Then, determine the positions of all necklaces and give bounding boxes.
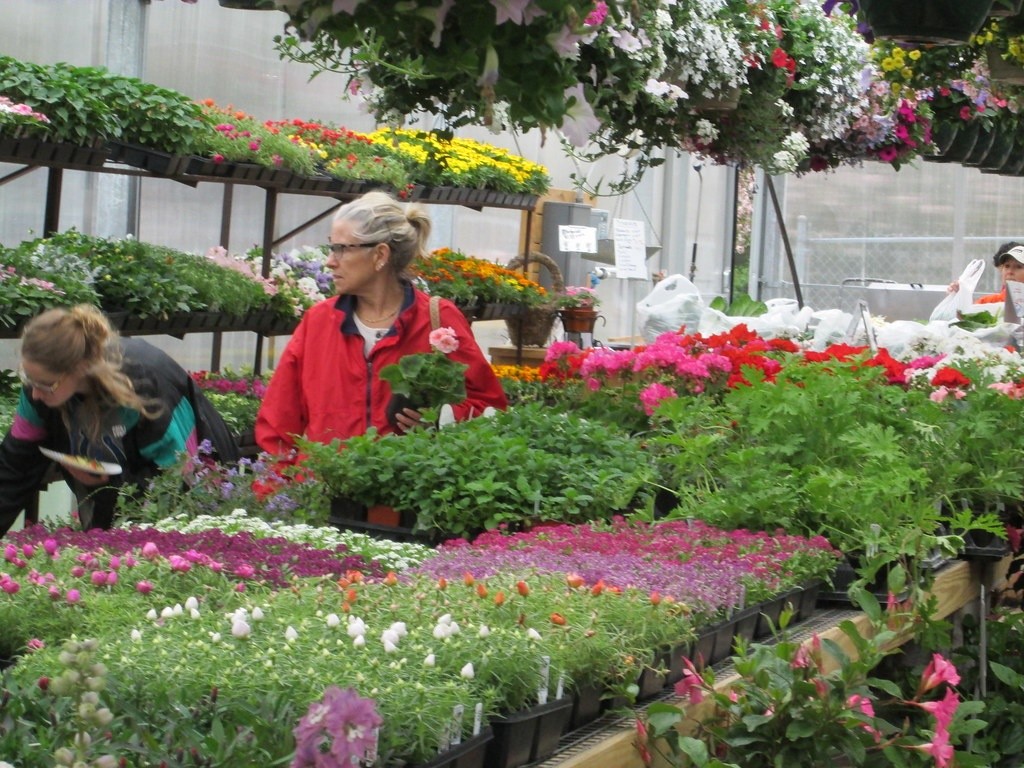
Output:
[364,289,403,323]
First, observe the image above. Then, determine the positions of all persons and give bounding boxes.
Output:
[250,190,510,503]
[0,303,245,542]
[948,241,1024,304]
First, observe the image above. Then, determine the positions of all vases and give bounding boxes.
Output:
[329,496,406,529]
[104,300,527,335]
[560,305,596,334]
[1,124,540,209]
[417,578,823,768]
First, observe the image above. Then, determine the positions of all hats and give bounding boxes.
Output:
[999,246,1024,264]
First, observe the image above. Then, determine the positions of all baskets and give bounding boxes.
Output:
[501,251,565,348]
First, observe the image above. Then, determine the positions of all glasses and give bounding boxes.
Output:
[15,360,72,396]
[318,242,399,261]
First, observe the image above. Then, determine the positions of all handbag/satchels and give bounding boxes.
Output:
[927,260,986,320]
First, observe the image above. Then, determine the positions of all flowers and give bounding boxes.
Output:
[0,325,1024,768]
[555,285,600,311]
[1,55,552,201]
[1,229,548,326]
